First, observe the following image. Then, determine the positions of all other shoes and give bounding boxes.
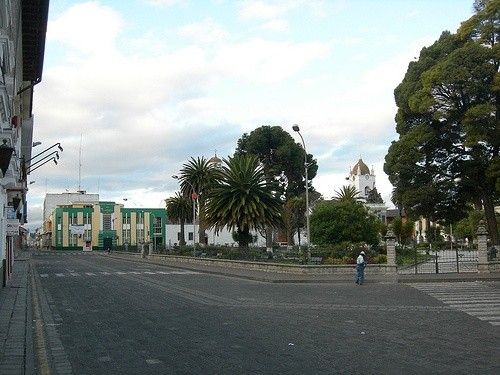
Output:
[360,283,362,285]
[356,281,359,284]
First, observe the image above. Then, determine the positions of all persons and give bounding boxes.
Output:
[355,251,366,285]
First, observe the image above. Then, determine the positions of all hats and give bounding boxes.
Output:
[359,251,365,255]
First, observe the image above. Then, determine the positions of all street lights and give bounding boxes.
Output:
[171,175,197,257]
[292,124,312,263]
[122,198,141,252]
